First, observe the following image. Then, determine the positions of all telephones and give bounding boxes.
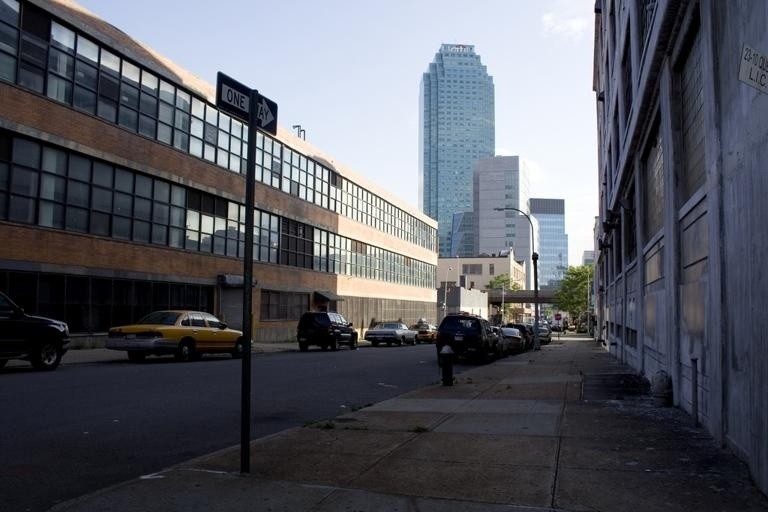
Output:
[212,68,280,138]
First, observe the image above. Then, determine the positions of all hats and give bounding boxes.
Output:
[491,311,576,356]
[407,318,437,342]
[104,307,255,364]
[364,321,419,347]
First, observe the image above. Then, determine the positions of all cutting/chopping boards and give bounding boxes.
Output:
[437,345,457,386]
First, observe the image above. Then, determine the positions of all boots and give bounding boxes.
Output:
[293,309,363,353]
[0,289,72,374]
[433,311,501,366]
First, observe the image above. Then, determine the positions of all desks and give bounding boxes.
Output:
[493,207,542,353]
[501,280,507,321]
[442,263,456,320]
[585,270,591,336]
